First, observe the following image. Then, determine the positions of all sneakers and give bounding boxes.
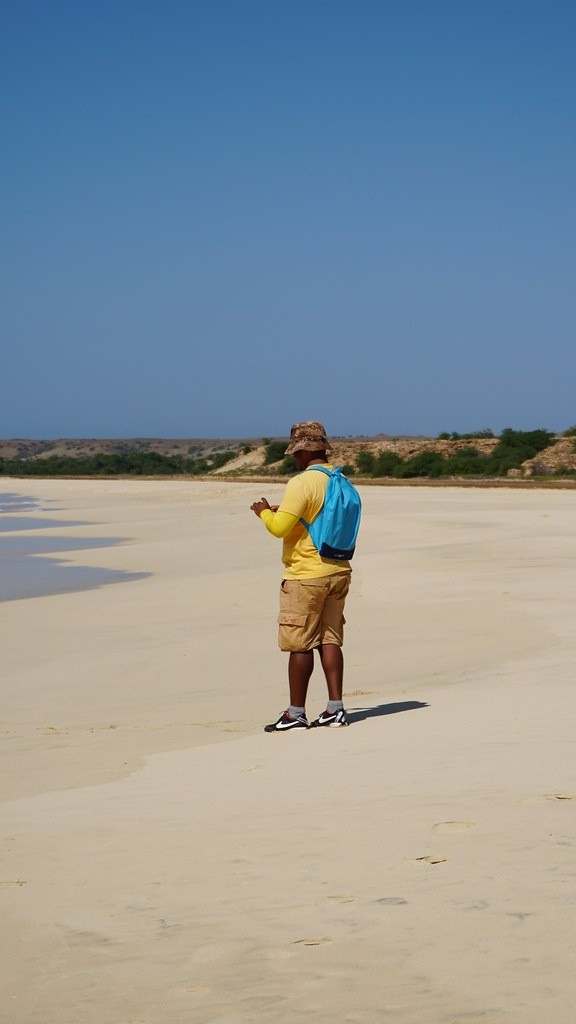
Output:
[309,708,348,729]
[264,710,308,734]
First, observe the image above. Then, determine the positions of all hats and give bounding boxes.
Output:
[284,421,334,455]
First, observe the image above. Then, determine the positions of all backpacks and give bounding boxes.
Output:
[299,466,361,560]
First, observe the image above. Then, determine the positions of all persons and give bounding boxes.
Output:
[250,420,353,732]
